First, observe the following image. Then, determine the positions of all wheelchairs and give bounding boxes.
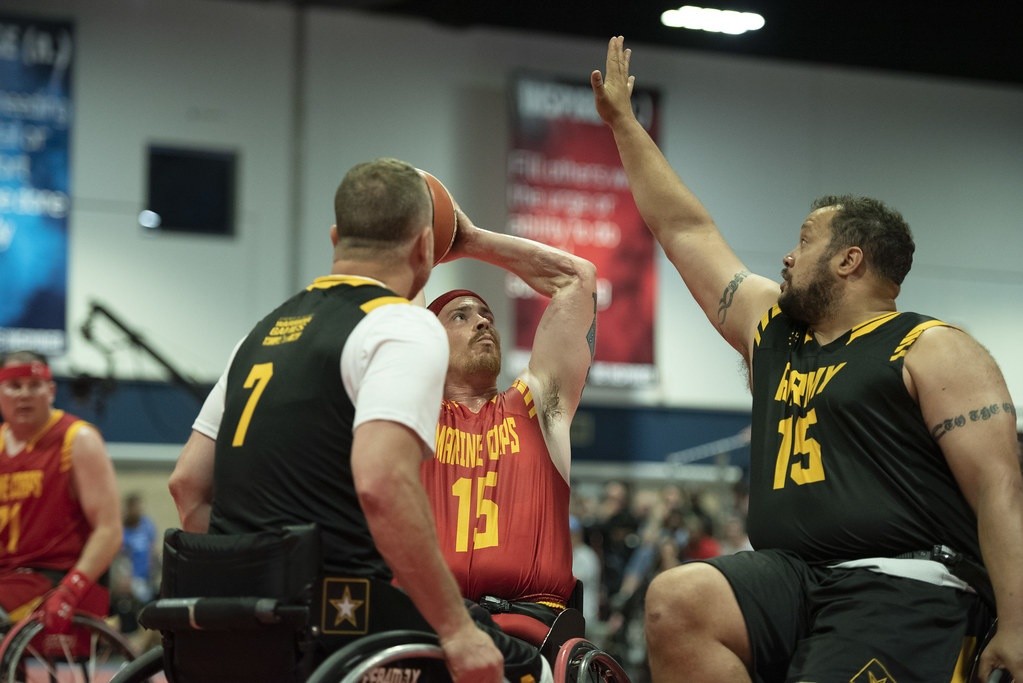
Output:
[0,577,1013,683]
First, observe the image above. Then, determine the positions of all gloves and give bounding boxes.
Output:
[32,568,93,636]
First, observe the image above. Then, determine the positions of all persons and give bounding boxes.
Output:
[390,196,599,643]
[567,480,756,682]
[118,494,160,604]
[0,350,125,683]
[167,158,556,683]
[592,37,1022,683]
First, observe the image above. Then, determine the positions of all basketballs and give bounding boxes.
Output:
[417,168,457,269]
